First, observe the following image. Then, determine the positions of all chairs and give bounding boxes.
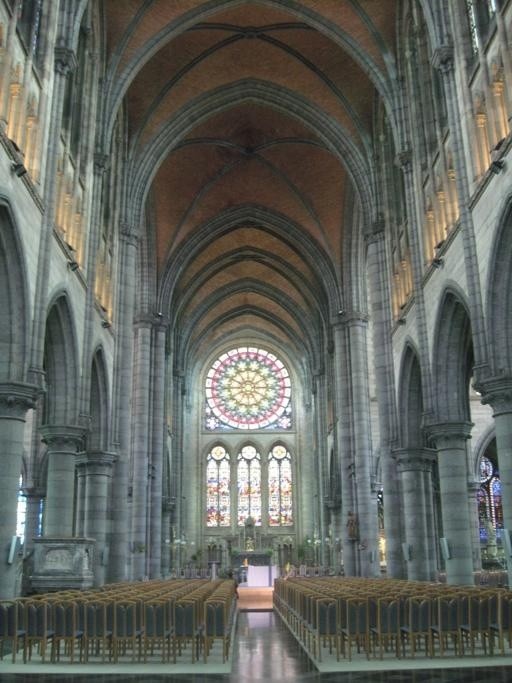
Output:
[270,573,512,661]
[0,578,239,664]
